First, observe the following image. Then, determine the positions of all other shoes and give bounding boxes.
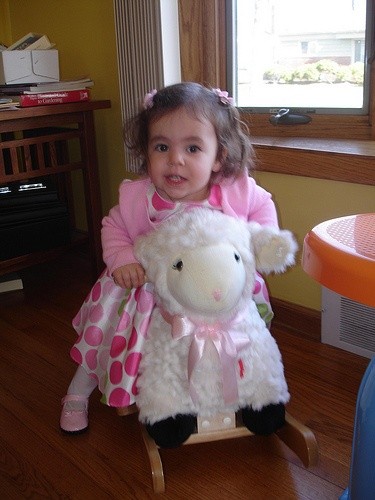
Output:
[60,393,88,431]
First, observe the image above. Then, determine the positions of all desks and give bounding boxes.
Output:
[2,99,109,286]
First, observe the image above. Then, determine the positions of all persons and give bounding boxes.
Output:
[61,83,280,433]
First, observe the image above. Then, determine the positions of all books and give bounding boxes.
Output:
[0,76,94,112]
